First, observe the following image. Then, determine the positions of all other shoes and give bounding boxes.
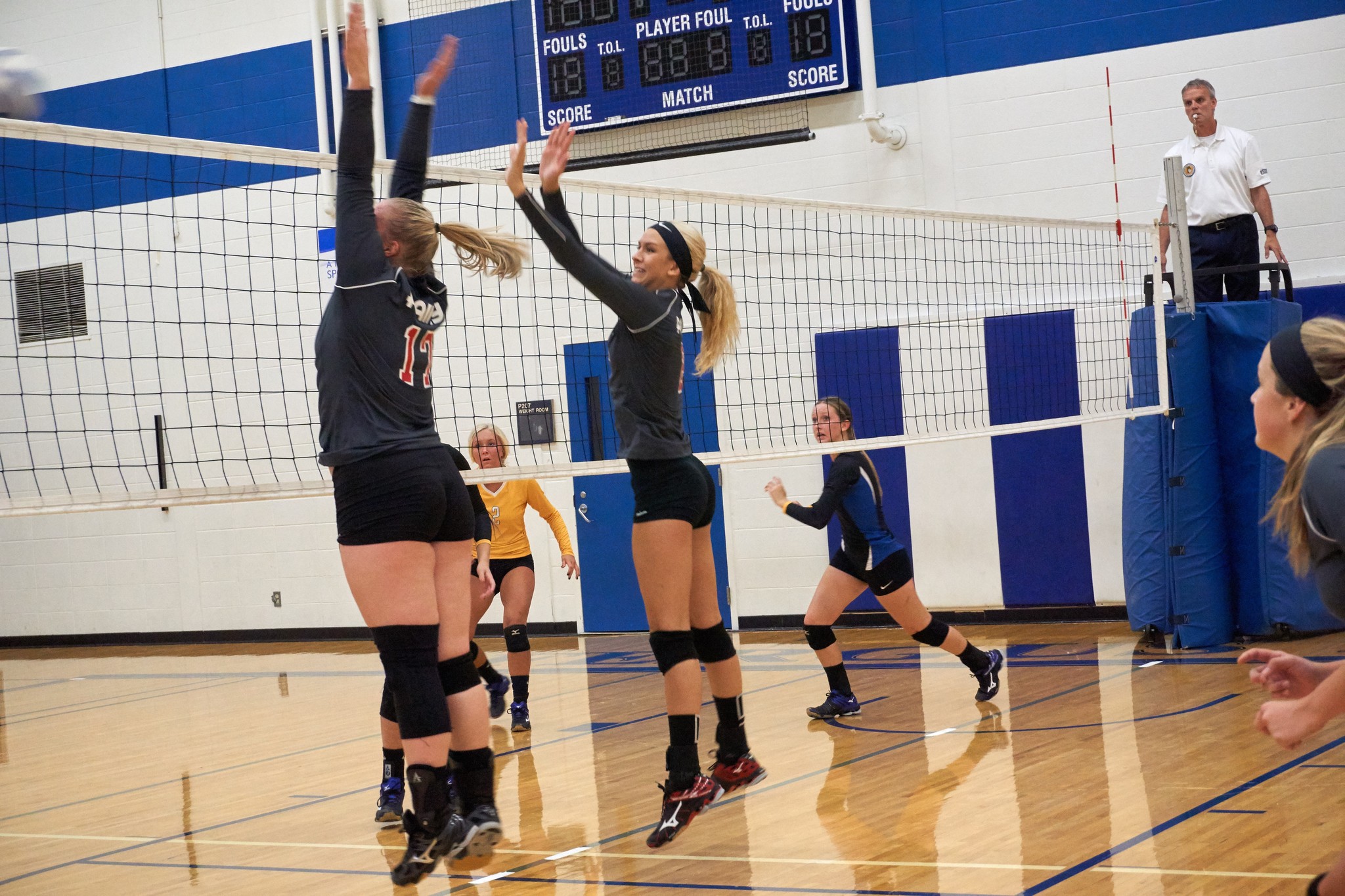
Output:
[377,827,408,862]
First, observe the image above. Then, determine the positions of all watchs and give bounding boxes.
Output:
[1264,224,1278,234]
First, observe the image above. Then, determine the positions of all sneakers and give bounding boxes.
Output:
[706,748,768,793]
[390,809,478,887]
[490,725,532,752]
[975,700,1009,750]
[647,771,726,849]
[968,649,1005,701]
[807,716,862,739]
[507,702,531,731]
[485,675,511,718]
[374,777,404,822]
[806,689,861,718]
[447,806,503,871]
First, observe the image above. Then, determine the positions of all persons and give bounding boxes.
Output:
[313,1,500,882]
[764,396,1003,719]
[469,423,581,733]
[506,118,742,850]
[1159,78,1289,303]
[1235,314,1345,896]
[374,443,496,822]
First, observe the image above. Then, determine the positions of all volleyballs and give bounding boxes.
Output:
[0,47,44,117]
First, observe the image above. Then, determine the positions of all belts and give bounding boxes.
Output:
[1207,219,1229,231]
